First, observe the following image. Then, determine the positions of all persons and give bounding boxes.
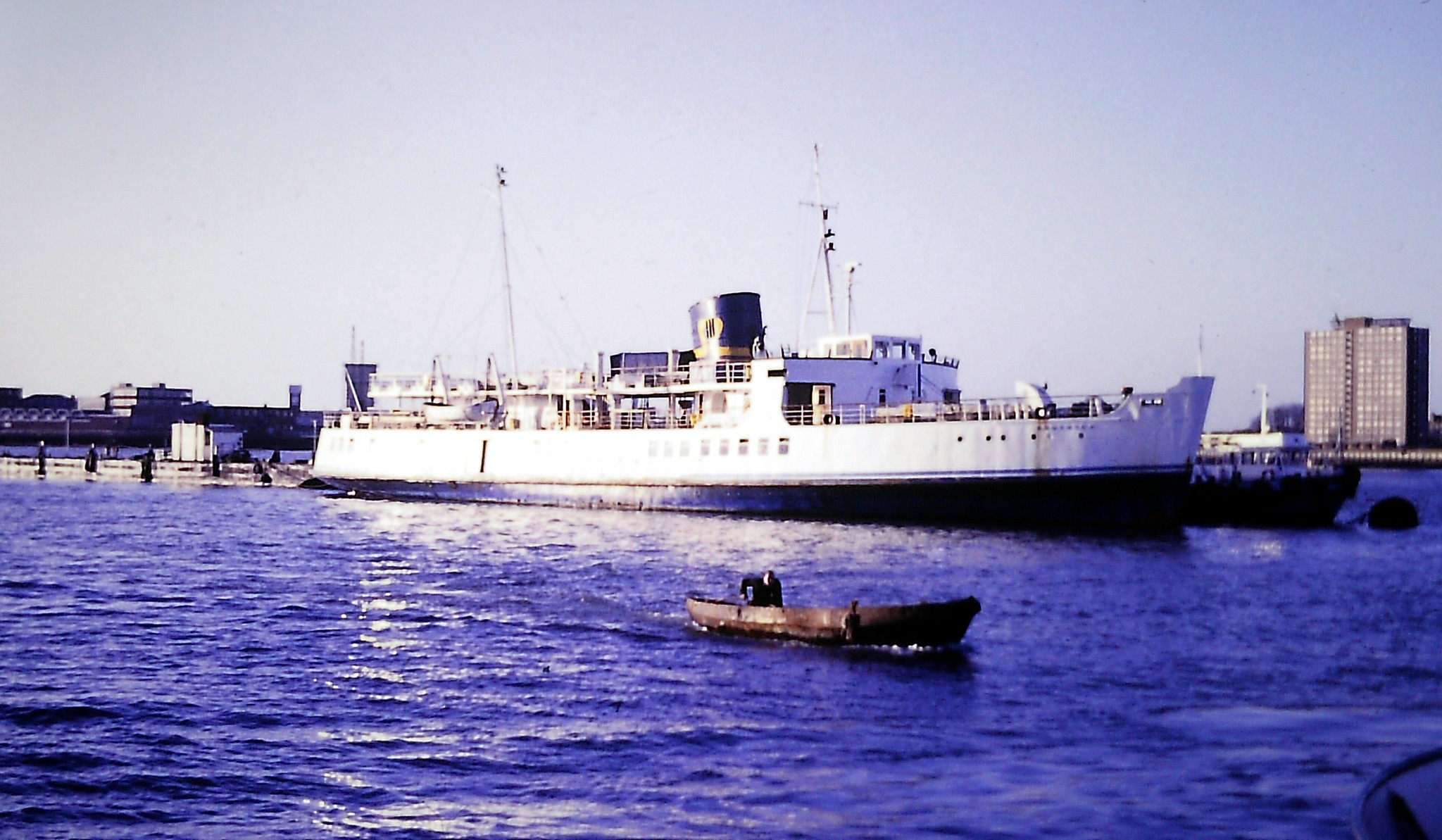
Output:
[739,569,784,609]
[1188,448,1353,494]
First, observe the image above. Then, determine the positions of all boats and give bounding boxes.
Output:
[687,595,981,648]
[1189,381,1362,531]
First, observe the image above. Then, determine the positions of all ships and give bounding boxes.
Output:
[306,147,1217,535]
